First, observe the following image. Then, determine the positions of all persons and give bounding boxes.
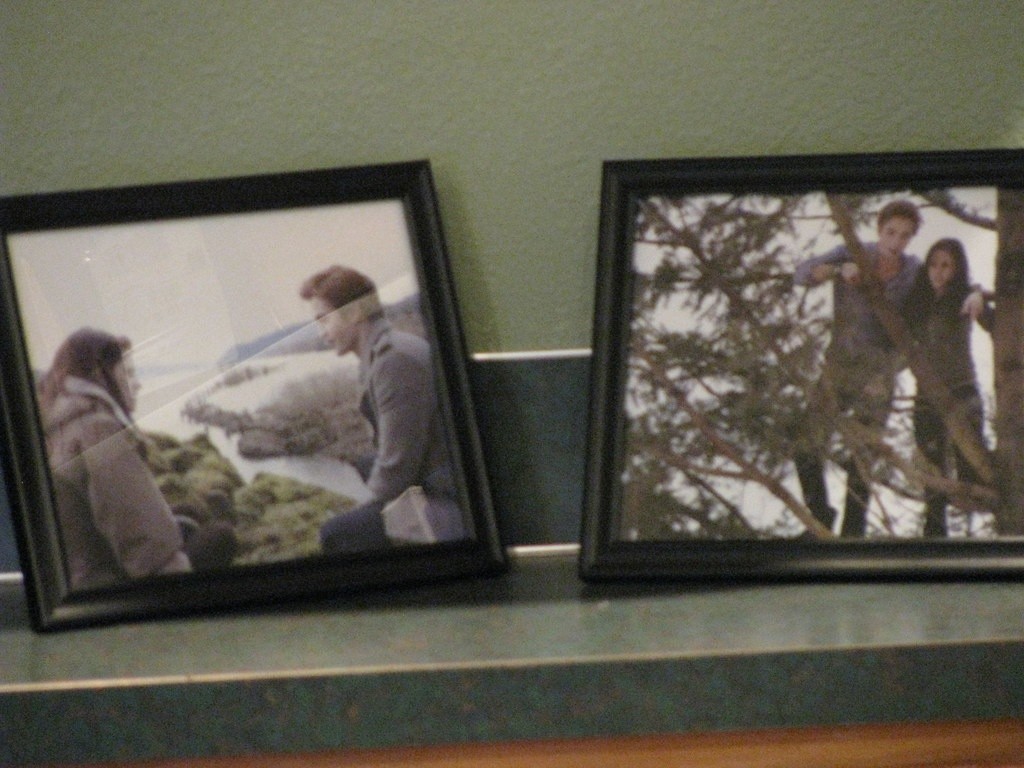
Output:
[37,327,237,589]
[793,201,994,538]
[300,265,466,553]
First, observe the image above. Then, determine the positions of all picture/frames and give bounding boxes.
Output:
[570,142,1024,591]
[0,153,519,639]
